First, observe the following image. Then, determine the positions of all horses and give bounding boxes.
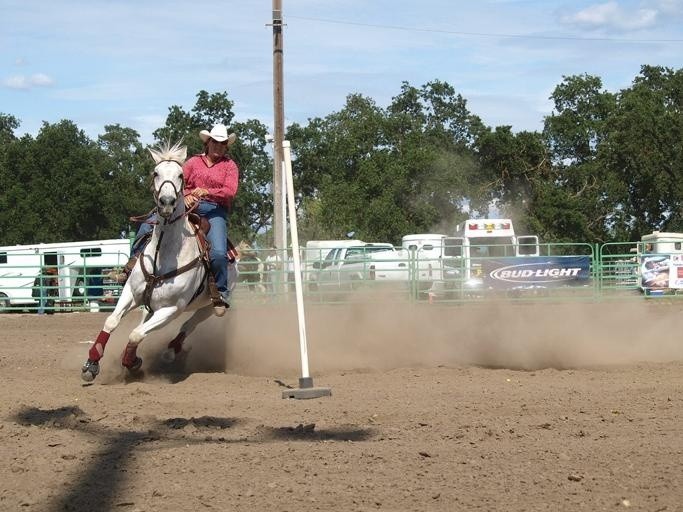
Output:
[80,135,238,382]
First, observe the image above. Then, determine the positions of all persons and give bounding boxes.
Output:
[110,122,239,318]
[472,247,486,264]
[251,243,301,293]
[32,267,58,315]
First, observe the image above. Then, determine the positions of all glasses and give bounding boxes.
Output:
[210,138,230,146]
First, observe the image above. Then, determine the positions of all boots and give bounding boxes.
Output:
[107,255,138,284]
[211,289,228,318]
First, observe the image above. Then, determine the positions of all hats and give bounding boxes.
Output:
[197,123,237,148]
